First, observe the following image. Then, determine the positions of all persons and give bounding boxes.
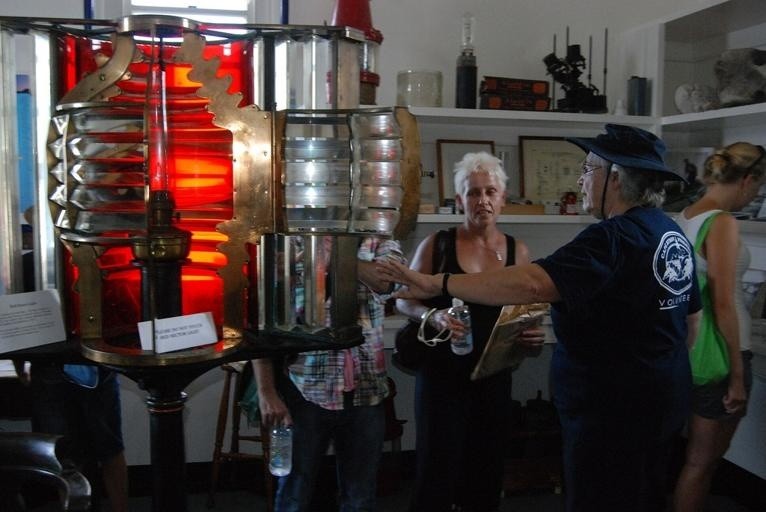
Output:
[8,356,131,512]
[673,139,766,512]
[248,229,407,512]
[395,147,547,512]
[373,120,704,510]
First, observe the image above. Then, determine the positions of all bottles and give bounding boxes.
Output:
[562,190,577,215]
[418,193,434,214]
[266,424,293,479]
[396,70,441,106]
[445,297,474,356]
[445,199,454,215]
[453,11,478,110]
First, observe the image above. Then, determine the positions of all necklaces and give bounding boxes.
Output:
[460,224,504,262]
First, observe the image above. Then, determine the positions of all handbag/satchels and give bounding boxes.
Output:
[239,362,281,425]
[684,274,730,387]
[391,317,430,376]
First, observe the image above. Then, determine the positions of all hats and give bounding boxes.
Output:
[566,124,691,186]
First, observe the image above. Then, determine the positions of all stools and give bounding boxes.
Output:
[210,358,402,512]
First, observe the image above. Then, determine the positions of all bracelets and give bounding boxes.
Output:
[440,273,454,301]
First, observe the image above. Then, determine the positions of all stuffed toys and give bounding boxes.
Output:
[672,48,766,115]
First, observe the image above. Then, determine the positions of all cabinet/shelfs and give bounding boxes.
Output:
[380,104,654,353]
[654,0,765,377]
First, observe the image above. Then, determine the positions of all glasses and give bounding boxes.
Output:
[743,145,766,180]
[417,307,453,346]
[583,163,610,173]
